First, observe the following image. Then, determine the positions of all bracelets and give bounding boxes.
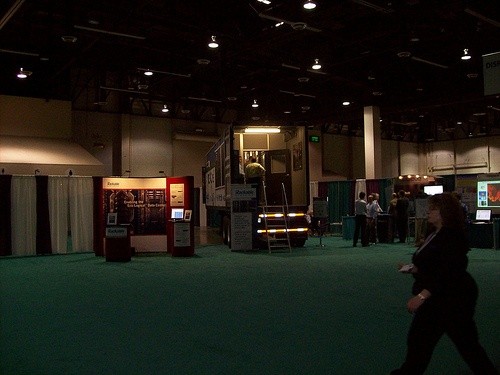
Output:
[418,293,425,300]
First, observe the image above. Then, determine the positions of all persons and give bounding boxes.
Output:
[369,193,383,224]
[393,190,409,243]
[244,157,266,202]
[452,192,470,224]
[388,192,398,237]
[239,156,246,176]
[390,193,496,375]
[366,195,378,243]
[353,192,371,247]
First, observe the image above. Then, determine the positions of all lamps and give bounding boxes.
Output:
[311,58,321,70]
[206,34,219,48]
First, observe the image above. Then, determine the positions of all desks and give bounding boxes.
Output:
[341,216,357,239]
[407,217,428,247]
